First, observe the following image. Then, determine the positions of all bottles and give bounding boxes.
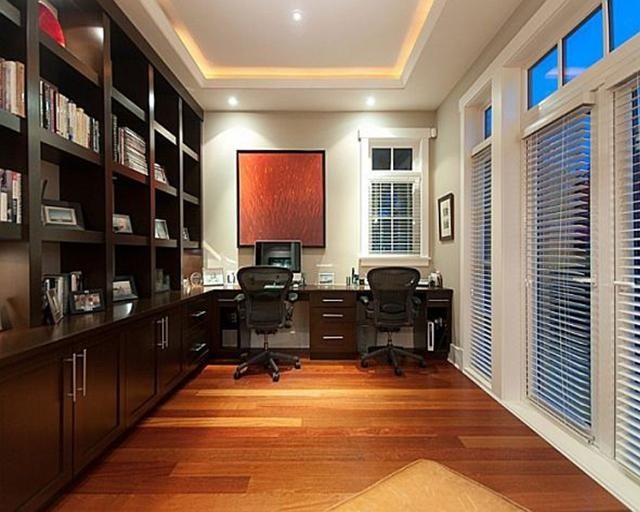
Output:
[436,271,443,287]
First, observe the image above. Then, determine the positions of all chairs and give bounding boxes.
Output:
[229,264,301,384]
[360,268,430,376]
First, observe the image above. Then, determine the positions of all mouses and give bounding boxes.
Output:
[293,283,299,288]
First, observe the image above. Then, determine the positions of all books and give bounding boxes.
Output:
[1,53,169,223]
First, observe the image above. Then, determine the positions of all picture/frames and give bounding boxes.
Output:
[235,149,327,249]
[317,272,335,286]
[202,265,225,287]
[438,193,454,241]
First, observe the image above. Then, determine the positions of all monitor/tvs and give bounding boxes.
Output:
[253,240,302,285]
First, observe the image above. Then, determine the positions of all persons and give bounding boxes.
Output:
[193,274,200,286]
[41,278,53,324]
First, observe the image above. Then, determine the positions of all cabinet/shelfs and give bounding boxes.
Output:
[0,0,216,510]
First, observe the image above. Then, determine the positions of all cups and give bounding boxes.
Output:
[429,276,435,288]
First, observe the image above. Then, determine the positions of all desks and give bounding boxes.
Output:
[215,284,454,362]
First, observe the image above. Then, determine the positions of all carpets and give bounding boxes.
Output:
[315,458,531,512]
